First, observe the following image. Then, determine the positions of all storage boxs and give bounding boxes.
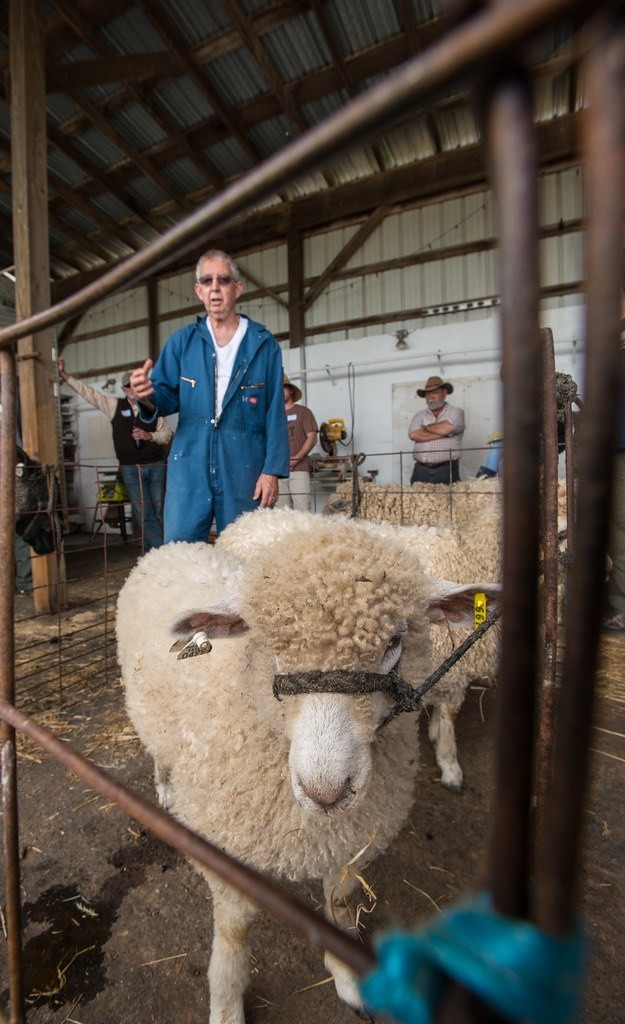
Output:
[98,482,130,501]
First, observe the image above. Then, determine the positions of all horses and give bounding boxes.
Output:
[14,446,63,555]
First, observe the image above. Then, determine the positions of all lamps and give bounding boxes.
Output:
[395,328,409,349]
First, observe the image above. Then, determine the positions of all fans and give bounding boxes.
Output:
[319,422,347,459]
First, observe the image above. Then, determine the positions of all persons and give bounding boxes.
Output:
[128,251,290,547]
[58,357,173,549]
[408,364,505,487]
[274,373,318,512]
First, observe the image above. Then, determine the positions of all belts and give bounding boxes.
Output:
[418,460,458,469]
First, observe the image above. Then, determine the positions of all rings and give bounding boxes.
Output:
[273,497,276,499]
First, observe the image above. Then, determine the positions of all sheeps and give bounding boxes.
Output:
[112,477,568,1024]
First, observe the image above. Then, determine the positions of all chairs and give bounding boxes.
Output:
[90,464,133,546]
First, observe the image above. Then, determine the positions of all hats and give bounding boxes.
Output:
[122,373,132,387]
[417,377,453,397]
[284,374,303,400]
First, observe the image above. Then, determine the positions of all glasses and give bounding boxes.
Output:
[124,382,131,388]
[198,274,237,287]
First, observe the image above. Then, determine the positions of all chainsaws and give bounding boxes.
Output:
[318,418,347,456]
